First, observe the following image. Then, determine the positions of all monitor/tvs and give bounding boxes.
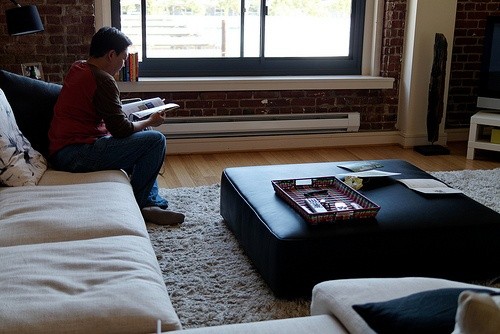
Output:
[476,15,500,113]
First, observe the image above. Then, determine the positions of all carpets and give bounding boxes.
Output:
[146,167,500,330]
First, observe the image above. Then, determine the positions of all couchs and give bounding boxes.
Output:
[0,165,500,334]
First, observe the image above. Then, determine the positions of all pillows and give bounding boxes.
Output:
[0,88,44,187]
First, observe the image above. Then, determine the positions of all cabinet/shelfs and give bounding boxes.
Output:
[466,109,500,161]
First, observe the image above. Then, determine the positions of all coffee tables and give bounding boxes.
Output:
[216,157,500,295]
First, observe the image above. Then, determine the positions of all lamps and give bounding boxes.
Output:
[5,0,46,37]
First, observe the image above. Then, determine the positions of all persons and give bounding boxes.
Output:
[47,27,185,227]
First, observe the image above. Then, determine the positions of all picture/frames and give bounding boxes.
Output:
[20,61,45,81]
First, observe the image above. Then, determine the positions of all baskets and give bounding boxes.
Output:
[271,176,381,224]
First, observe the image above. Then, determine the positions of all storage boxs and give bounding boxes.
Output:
[490,129,500,144]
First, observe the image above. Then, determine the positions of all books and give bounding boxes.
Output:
[121,97,180,122]
[114,52,138,81]
